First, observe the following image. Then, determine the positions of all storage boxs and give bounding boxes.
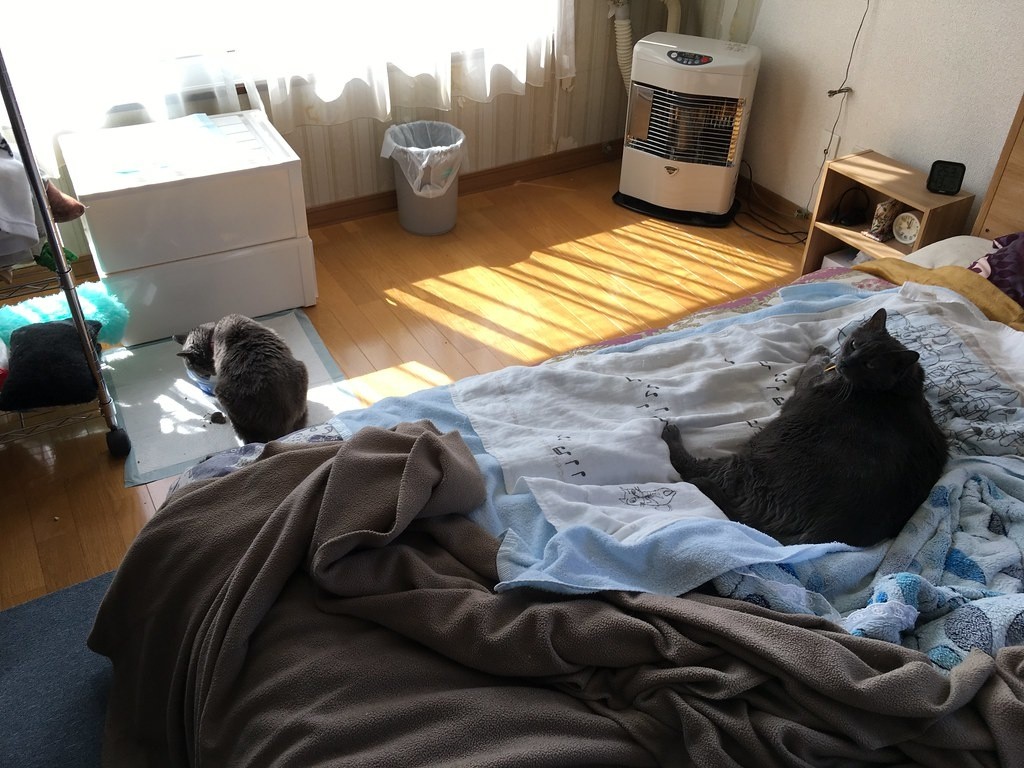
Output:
[57,108,307,271]
[821,250,855,276]
[77,218,317,348]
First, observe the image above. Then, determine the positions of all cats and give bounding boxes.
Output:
[172,312,308,446]
[659,306,950,549]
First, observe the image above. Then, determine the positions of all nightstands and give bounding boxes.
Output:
[801,148,975,297]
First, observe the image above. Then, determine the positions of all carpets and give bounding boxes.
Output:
[99,306,362,491]
[0,570,121,768]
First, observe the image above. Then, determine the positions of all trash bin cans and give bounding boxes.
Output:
[382,121,468,237]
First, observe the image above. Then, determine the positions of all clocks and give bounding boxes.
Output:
[894,211,923,244]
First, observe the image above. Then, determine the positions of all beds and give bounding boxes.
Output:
[88,87,1024,768]
